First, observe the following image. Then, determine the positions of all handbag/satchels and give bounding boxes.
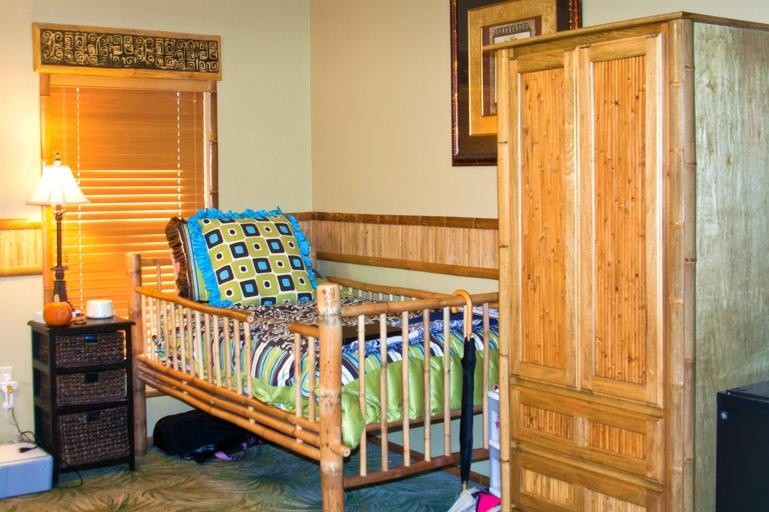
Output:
[152,408,266,465]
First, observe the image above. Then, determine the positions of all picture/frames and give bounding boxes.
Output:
[449,0,585,166]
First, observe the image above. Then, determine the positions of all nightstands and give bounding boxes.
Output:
[28,313,138,483]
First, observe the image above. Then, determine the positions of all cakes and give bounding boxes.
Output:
[86,300,112,318]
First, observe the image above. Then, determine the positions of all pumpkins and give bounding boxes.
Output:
[43,295,72,326]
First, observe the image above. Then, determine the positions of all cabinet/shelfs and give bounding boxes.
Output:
[486,12,769,511]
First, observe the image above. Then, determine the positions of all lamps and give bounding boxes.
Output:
[25,153,93,323]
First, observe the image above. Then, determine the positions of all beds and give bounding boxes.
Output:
[128,251,500,511]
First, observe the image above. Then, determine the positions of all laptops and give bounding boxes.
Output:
[342,323,410,344]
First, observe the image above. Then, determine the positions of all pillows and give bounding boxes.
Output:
[166,204,327,312]
